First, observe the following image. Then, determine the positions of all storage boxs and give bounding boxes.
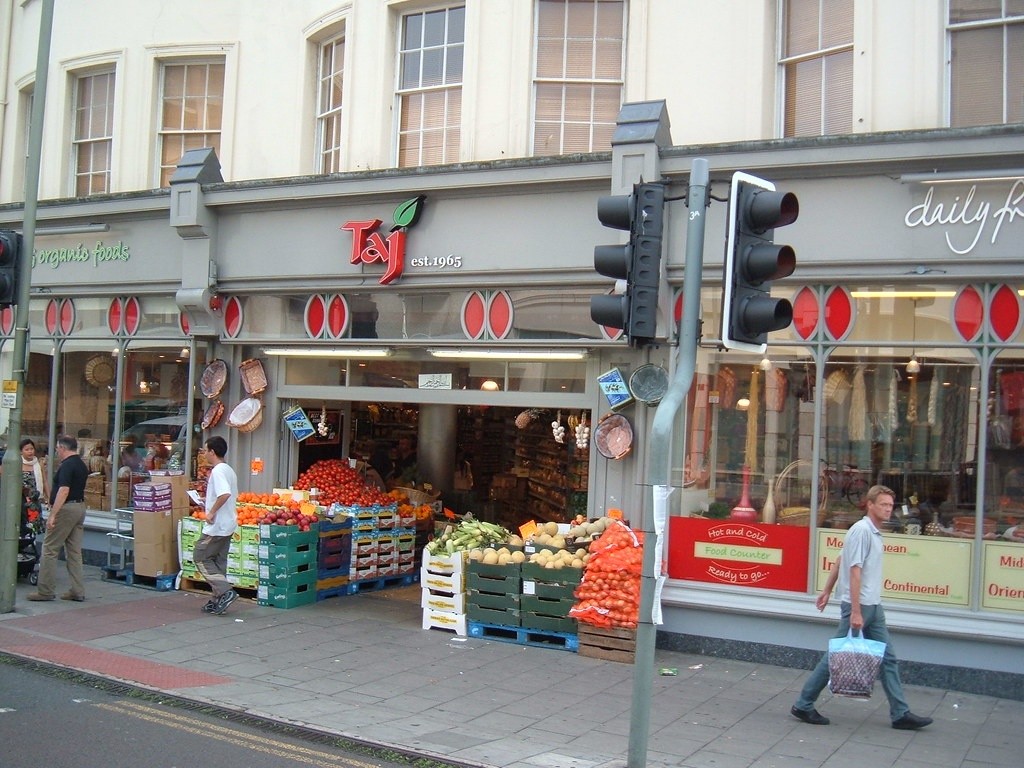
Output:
[135,476,637,664]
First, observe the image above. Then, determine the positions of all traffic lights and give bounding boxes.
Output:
[590,173,666,346]
[721,171,797,355]
[0,230,23,309]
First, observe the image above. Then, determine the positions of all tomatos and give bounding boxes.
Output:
[293,458,395,507]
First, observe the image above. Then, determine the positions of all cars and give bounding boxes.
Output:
[115,415,187,455]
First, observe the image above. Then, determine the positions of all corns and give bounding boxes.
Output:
[428,518,513,556]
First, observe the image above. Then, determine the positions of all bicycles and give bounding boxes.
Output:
[809,458,872,509]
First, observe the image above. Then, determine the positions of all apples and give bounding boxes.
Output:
[260,507,317,532]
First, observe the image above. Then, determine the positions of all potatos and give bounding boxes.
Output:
[575,524,646,628]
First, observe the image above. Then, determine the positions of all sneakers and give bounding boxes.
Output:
[27,593,56,601]
[200,600,227,613]
[211,589,239,615]
[60,592,84,601]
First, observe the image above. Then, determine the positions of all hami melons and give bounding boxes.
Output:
[469,518,618,569]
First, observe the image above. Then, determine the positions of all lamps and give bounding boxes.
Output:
[427,347,591,360]
[180,348,190,358]
[481,377,499,391]
[260,345,395,356]
[760,349,772,370]
[736,384,750,411]
[906,297,920,372]
[111,348,119,356]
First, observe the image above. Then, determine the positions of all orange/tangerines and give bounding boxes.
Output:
[191,492,308,526]
[386,489,431,519]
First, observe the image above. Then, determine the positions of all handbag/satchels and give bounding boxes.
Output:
[828,628,887,701]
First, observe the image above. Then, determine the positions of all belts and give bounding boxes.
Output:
[64,498,84,504]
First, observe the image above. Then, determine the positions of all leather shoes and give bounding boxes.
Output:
[892,711,932,729]
[791,705,830,725]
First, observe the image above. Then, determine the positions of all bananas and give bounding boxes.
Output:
[568,415,578,430]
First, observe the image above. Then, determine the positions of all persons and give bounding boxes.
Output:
[375,438,417,478]
[27,436,87,603]
[18,438,50,551]
[193,436,239,615]
[77,428,143,505]
[999,462,1024,498]
[792,485,933,731]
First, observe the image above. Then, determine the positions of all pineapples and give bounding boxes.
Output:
[515,408,547,429]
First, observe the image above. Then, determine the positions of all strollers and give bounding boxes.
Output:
[16,518,40,586]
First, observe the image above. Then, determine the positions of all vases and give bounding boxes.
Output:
[731,466,757,521]
[763,479,775,524]
[925,512,944,536]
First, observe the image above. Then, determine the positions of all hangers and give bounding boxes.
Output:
[753,364,759,373]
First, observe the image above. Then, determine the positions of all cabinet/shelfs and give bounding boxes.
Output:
[349,419,591,522]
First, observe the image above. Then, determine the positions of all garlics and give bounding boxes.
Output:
[317,423,328,436]
[552,421,565,443]
[574,423,589,449]
